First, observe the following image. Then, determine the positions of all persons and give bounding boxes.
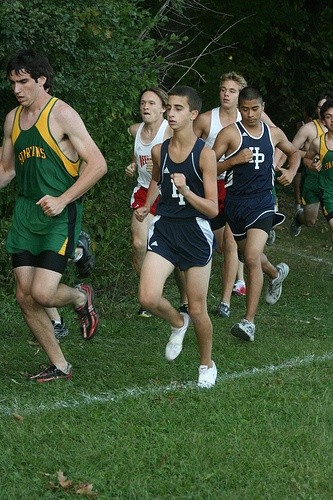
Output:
[0,49,109,380]
[193,72,282,315]
[125,86,189,317]
[266,96,333,246]
[137,87,218,388]
[212,85,302,340]
[26,232,94,346]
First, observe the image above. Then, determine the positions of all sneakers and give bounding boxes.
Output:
[164,312,190,361]
[30,363,72,382]
[177,303,189,313]
[232,283,247,296]
[74,230,93,279]
[216,304,231,317]
[289,208,304,238]
[265,263,289,305]
[137,306,152,317]
[74,284,100,339]
[266,229,275,246]
[26,316,68,345]
[230,318,256,342]
[197,360,217,389]
